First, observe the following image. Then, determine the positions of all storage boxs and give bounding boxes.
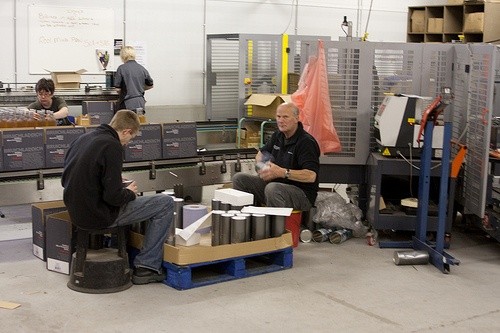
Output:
[81,99,122,124]
[43,68,87,90]
[32,200,104,275]
[0,121,198,173]
[243,92,292,118]
[406,0,500,45]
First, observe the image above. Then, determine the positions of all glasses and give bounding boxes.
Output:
[37,93,53,98]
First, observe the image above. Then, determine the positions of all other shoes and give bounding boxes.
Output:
[132,267,167,284]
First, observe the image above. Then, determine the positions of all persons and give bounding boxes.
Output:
[114,45,153,114]
[27,77,69,118]
[60,109,175,285]
[232,101,320,210]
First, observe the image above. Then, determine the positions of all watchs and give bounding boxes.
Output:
[284,168,290,179]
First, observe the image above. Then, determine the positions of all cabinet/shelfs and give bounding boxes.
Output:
[365,152,456,235]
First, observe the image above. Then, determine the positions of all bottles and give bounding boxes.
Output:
[0,107,56,128]
[136,107,146,124]
